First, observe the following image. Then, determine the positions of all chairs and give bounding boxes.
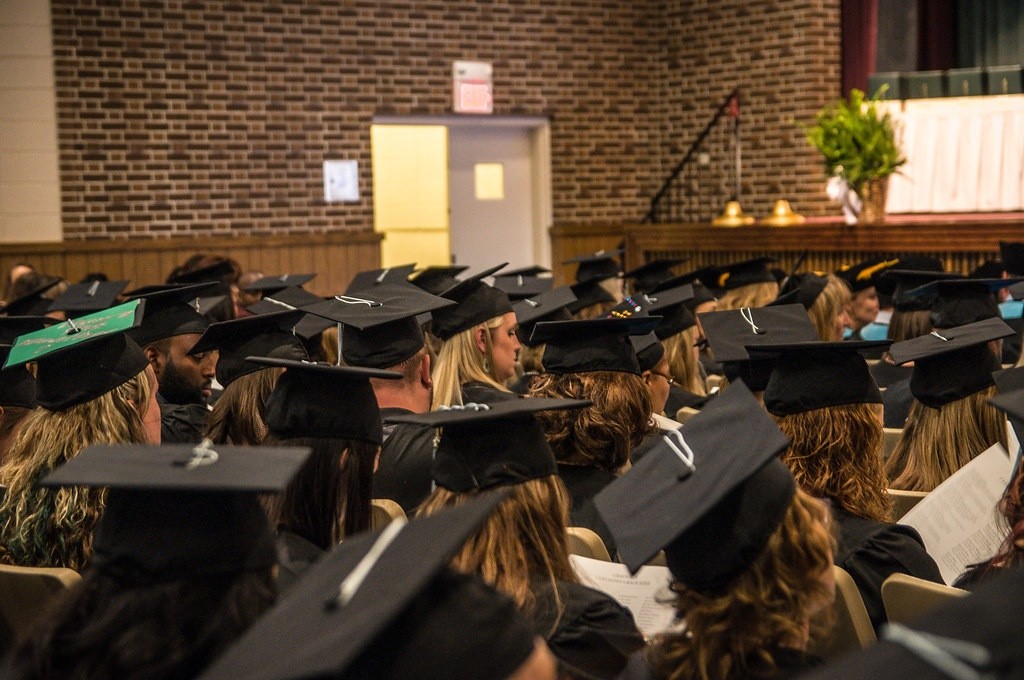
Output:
[0,374,971,680]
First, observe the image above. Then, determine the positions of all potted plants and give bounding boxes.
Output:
[807,89,902,221]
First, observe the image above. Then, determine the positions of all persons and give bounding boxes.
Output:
[0,239,1024,680]
